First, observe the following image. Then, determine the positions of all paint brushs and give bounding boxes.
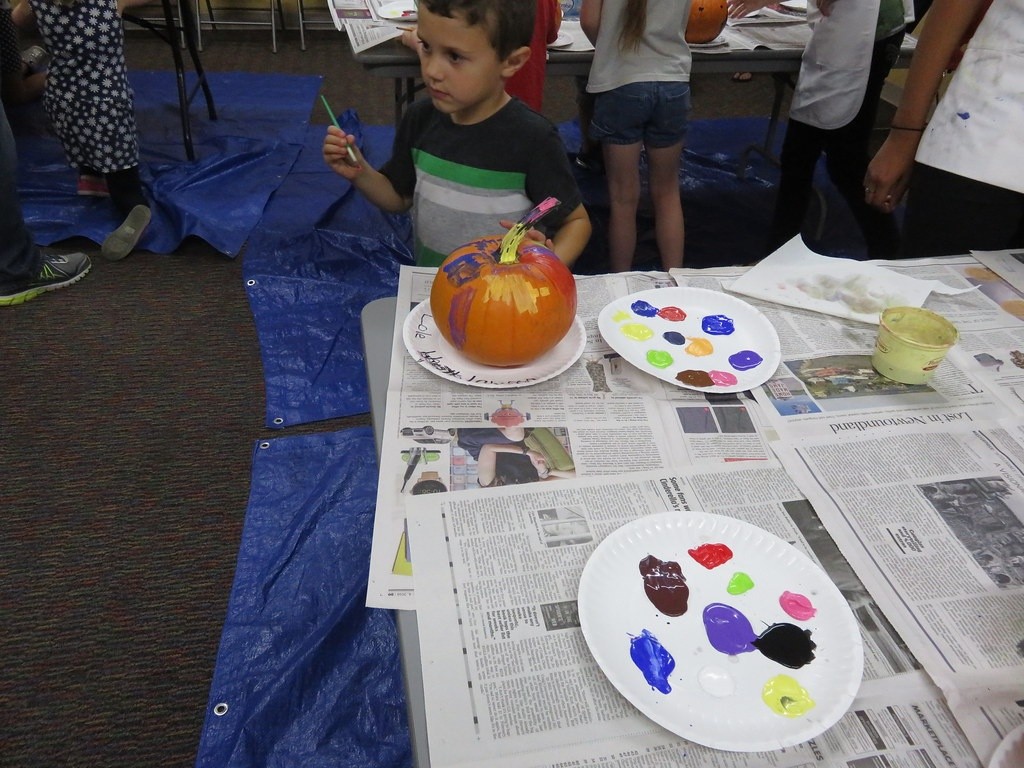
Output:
[321,95,362,167]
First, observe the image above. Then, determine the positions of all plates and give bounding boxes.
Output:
[401,295,588,390]
[546,31,574,46]
[686,36,727,47]
[984,721,1024,768]
[579,512,862,753]
[598,286,781,394]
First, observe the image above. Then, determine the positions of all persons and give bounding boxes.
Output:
[397,0,564,114]
[861,0,1024,258]
[579,0,695,272]
[0,0,154,305]
[727,0,917,261]
[453,424,577,488]
[322,0,594,269]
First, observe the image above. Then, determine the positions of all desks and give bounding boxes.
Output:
[357,251,1024,768]
[353,32,918,242]
[119,0,218,163]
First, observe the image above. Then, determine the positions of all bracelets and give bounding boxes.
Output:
[890,124,923,132]
[521,447,530,456]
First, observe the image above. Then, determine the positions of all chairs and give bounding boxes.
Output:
[123,0,185,50]
[195,0,285,54]
[296,0,337,51]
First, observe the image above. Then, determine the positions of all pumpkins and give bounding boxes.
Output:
[684,0,729,43]
[429,197,577,367]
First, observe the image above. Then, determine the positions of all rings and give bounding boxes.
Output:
[865,188,870,192]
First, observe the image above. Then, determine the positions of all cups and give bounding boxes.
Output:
[871,306,957,385]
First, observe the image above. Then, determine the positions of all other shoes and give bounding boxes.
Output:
[101,205,151,262]
[77,175,109,197]
[576,147,604,174]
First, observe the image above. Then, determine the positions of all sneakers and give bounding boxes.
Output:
[0,250,92,307]
